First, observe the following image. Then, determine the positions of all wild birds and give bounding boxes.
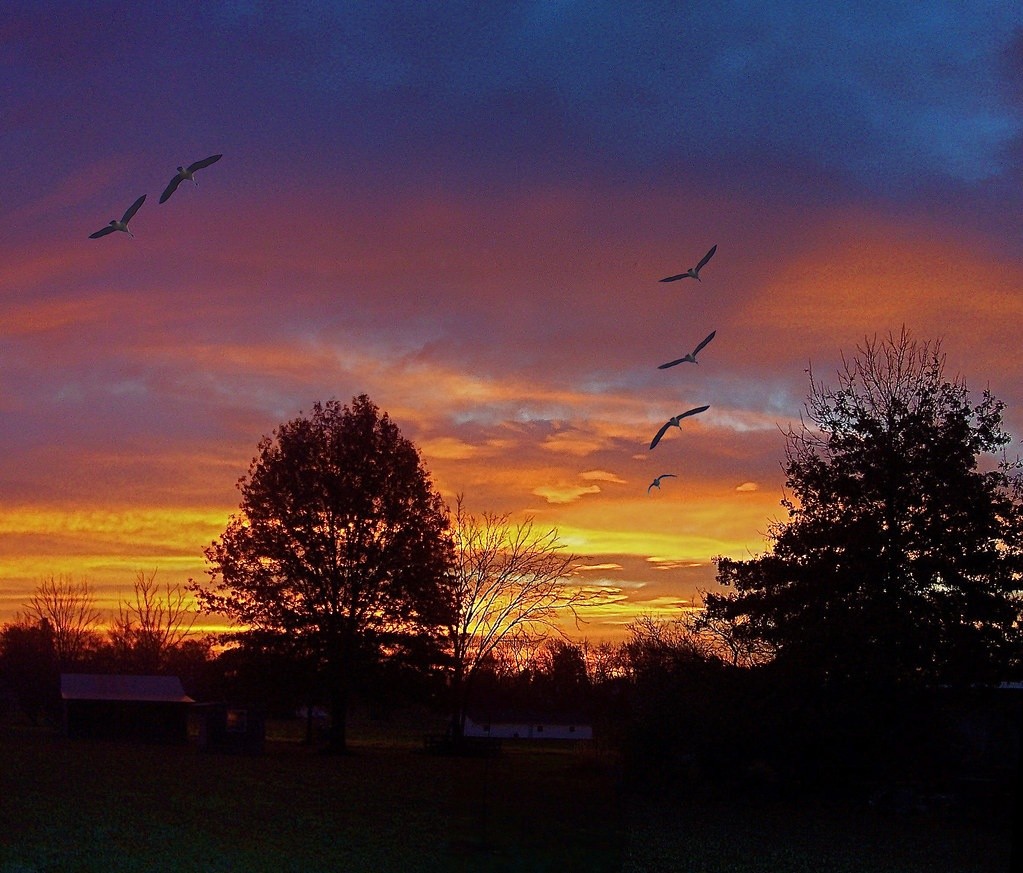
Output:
[650,405,712,449]
[89,194,147,239]
[658,244,717,282]
[658,330,716,369]
[648,474,677,493]
[159,154,223,204]
